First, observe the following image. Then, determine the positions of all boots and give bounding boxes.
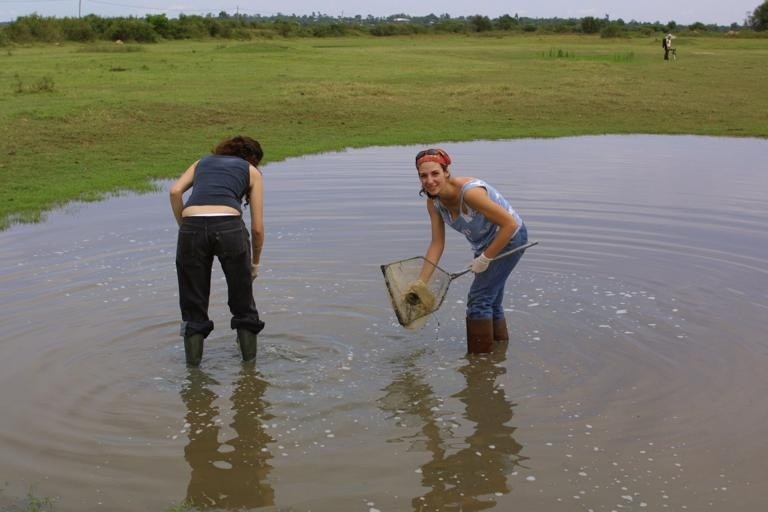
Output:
[237,328,258,362]
[465,315,495,357]
[183,333,204,369]
[493,318,509,342]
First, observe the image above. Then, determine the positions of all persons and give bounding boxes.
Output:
[167,134,265,365]
[400,147,530,352]
[664,33,676,61]
